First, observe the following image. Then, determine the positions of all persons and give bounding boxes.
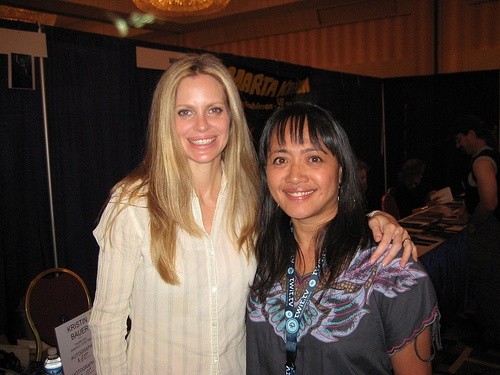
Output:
[456,117,500,349]
[246,102,444,375]
[89,53,417,375]
[356,159,425,223]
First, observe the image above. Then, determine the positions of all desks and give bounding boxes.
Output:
[398,199,470,355]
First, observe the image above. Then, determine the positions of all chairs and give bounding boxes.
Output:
[25,268,92,363]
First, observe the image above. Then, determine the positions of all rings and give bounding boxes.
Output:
[401,238,412,248]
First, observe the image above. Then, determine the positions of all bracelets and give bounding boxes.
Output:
[468,218,479,229]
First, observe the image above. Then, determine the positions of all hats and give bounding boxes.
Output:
[448,115,485,133]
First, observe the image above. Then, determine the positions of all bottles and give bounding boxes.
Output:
[43,347,63,375]
